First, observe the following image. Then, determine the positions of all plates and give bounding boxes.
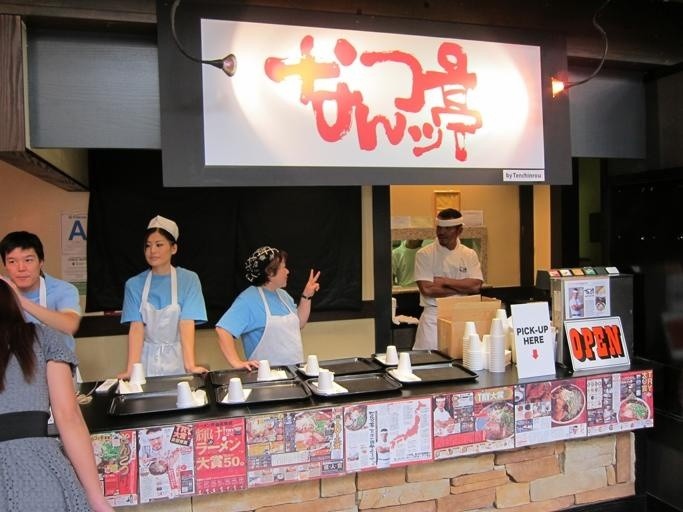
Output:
[109,389,211,415]
[115,374,206,395]
[373,350,454,366]
[210,365,297,385]
[306,373,402,396]
[294,357,382,377]
[385,363,479,383]
[216,380,312,404]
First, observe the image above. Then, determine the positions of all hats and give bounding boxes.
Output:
[147,216,179,241]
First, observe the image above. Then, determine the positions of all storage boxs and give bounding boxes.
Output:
[437,295,502,358]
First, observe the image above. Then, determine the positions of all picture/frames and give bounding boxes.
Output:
[433,192,462,222]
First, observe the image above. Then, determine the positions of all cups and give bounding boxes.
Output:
[306,355,319,374]
[398,352,411,376]
[228,378,243,401]
[258,360,270,379]
[463,309,509,373]
[317,372,333,391]
[386,346,398,363]
[176,382,194,403]
[130,363,146,384]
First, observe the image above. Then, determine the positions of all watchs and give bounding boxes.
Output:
[301,294,313,301]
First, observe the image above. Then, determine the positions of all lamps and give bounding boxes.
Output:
[551,18,608,96]
[170,1,238,76]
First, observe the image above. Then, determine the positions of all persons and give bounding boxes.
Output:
[0,280,119,512]
[569,287,584,318]
[117,215,209,381]
[460,239,481,261]
[434,397,454,437]
[1,231,83,396]
[375,429,395,469]
[215,247,320,372]
[142,428,179,466]
[412,209,482,351]
[392,238,423,328]
